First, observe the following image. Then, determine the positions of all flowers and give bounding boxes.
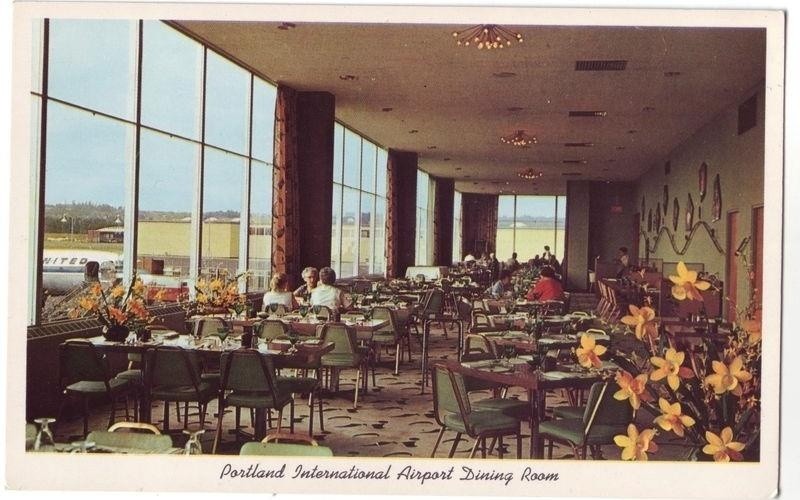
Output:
[575,262,760,462]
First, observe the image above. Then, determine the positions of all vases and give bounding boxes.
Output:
[691,446,760,462]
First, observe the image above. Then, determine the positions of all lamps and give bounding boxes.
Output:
[518,169,543,180]
[452,24,524,51]
[500,129,538,146]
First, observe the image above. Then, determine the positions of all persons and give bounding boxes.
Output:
[309,266,350,312]
[613,244,632,277]
[291,267,320,302]
[456,243,568,320]
[258,272,298,315]
